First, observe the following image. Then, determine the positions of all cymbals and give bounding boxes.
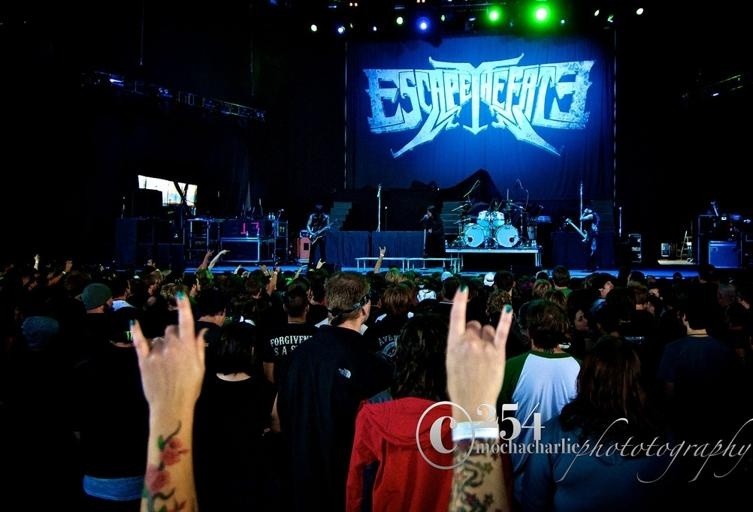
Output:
[454,218,471,224]
[452,203,467,211]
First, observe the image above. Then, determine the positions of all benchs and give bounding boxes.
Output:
[354,257,405,274]
[406,258,460,275]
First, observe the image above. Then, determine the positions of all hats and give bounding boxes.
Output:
[81,282,113,310]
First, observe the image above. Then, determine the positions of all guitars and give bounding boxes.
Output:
[566,218,590,245]
[307,219,338,245]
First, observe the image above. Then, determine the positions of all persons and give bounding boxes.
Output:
[1,248,753,512]
[487,197,507,218]
[305,204,330,263]
[581,207,601,272]
[418,206,444,261]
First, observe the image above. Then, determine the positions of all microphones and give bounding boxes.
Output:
[278,209,284,211]
[421,214,429,221]
[452,204,470,212]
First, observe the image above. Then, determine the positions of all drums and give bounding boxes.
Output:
[463,223,485,247]
[494,224,520,247]
[476,211,505,227]
